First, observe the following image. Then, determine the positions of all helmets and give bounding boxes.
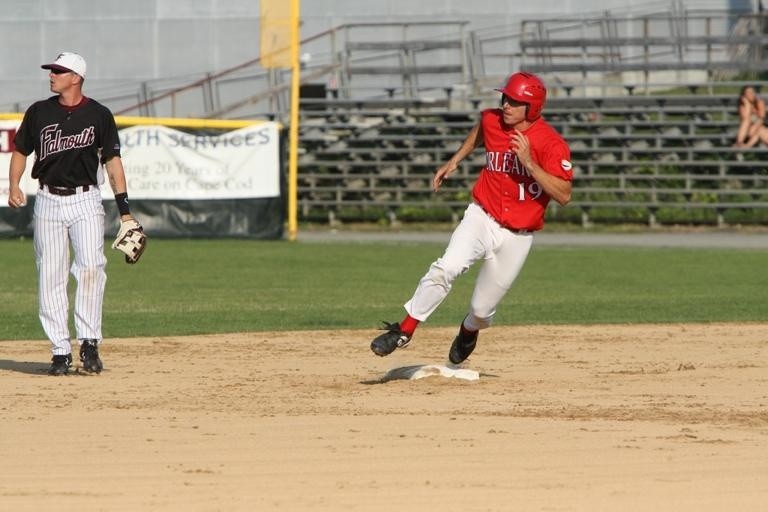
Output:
[493,72,546,121]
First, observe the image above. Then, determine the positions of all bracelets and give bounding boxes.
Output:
[115,192,130,216]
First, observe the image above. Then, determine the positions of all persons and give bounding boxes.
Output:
[8,52,133,376]
[732,85,768,148]
[370,72,573,364]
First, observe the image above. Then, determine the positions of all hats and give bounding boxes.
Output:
[41,52,86,79]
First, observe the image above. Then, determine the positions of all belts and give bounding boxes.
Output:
[39,181,89,196]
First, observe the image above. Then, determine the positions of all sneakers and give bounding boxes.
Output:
[80,341,103,375]
[48,353,72,376]
[449,313,479,364]
[370,323,413,357]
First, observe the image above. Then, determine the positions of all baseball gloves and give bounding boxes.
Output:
[111,219,147,264]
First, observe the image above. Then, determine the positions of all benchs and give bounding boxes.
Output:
[281,82,768,231]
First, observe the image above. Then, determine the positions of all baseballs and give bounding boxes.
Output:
[300,53,310,64]
